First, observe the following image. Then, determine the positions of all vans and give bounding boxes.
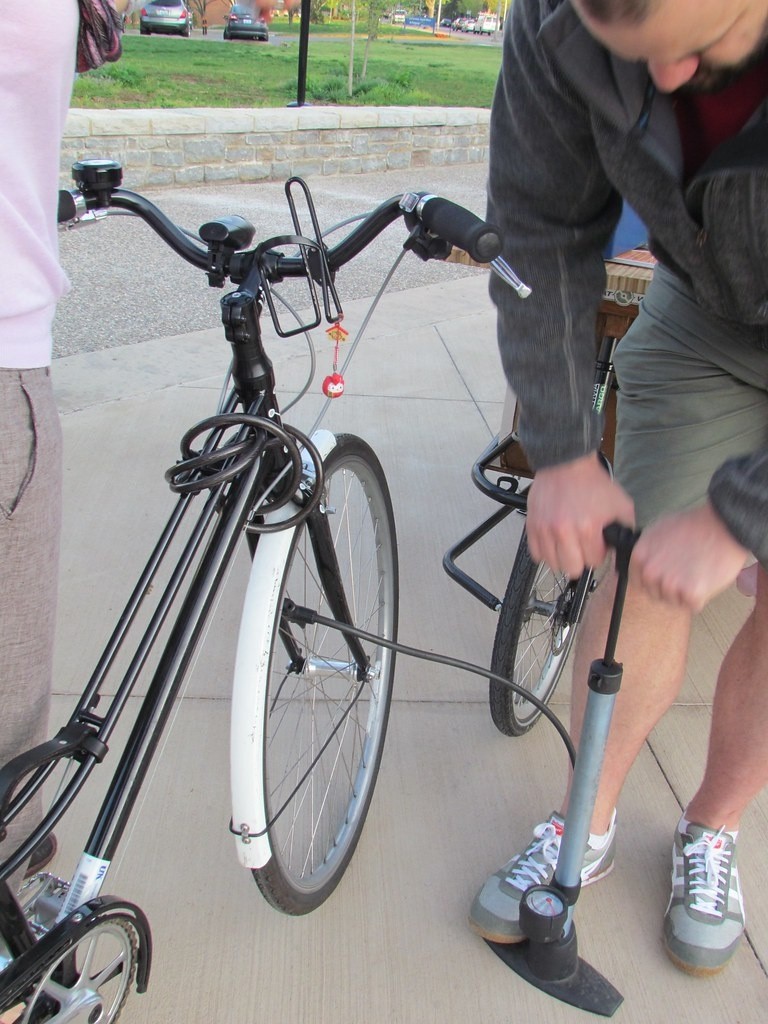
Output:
[392,10,407,25]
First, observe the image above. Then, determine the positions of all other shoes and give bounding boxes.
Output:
[21,831,58,880]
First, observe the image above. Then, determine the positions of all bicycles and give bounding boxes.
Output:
[0,157,532,1024]
[443,330,625,736]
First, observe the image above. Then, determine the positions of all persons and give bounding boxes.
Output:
[0,1,105,902]
[310,10,324,24]
[469,1,768,980]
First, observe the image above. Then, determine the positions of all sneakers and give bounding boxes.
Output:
[466,807,617,945]
[662,807,747,980]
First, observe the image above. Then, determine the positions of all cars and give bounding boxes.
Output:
[223,4,268,42]
[439,14,501,35]
[140,0,193,37]
